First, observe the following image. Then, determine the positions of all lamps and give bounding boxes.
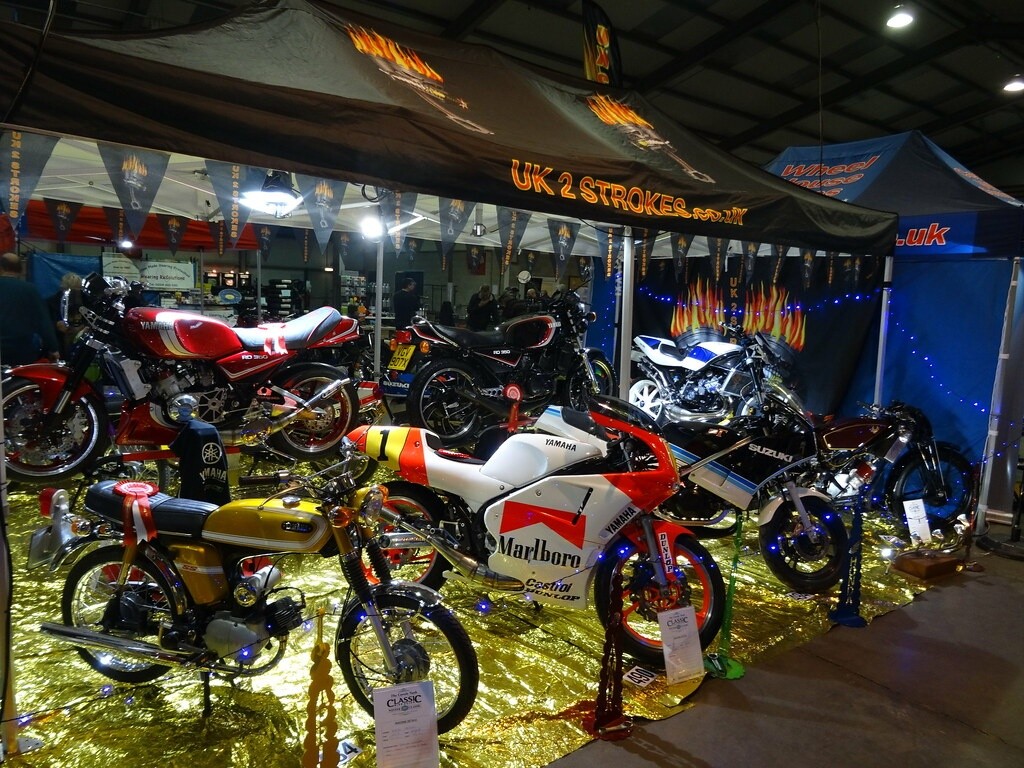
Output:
[240,170,304,218]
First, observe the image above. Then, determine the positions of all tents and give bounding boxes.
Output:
[582,128,1024,537]
[0,0,902,413]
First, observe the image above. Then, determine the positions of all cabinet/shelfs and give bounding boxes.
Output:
[366,292,392,311]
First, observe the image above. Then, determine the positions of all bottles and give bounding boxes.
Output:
[383,298,386,307]
[367,282,390,293]
[387,298,391,307]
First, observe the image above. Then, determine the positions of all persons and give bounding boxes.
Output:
[467,284,565,326]
[392,278,416,330]
[52,272,82,335]
[439,302,454,326]
[1,253,59,367]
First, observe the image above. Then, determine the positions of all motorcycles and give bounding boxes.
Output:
[379,263,619,450]
[345,394,727,667]
[625,318,977,595]
[0,270,364,485]
[24,432,481,738]
